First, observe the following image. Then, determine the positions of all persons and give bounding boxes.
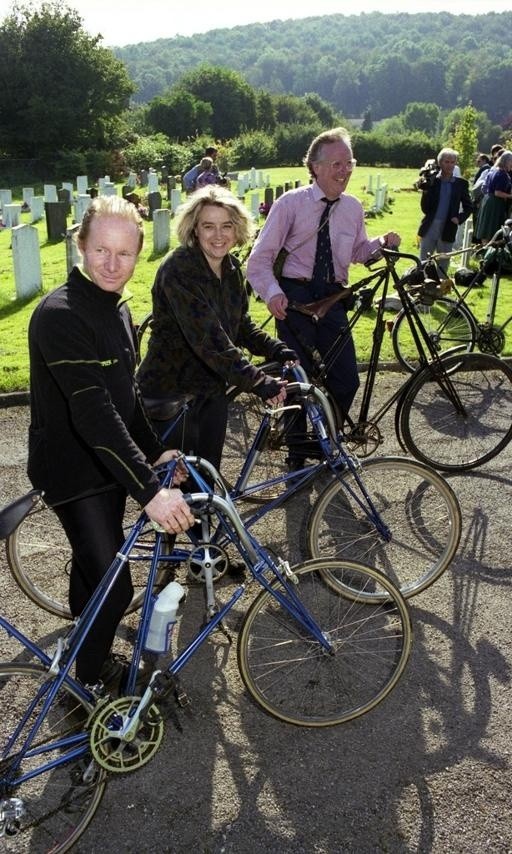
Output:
[23,193,200,727]
[137,183,303,581]
[243,127,401,461]
[417,147,476,282]
[197,156,221,186]
[182,147,219,194]
[451,142,511,277]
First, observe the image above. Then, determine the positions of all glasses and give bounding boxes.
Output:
[315,158,357,172]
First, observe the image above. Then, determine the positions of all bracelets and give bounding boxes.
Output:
[378,235,386,245]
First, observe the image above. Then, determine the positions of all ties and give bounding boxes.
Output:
[313,198,339,285]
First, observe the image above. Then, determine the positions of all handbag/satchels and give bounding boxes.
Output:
[248,246,290,302]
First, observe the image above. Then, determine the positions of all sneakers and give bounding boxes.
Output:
[68,651,152,722]
[189,549,246,576]
[287,441,332,485]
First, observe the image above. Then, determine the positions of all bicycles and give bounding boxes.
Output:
[390,225,512,377]
[208,244,512,506]
[0,451,412,854]
[0,357,463,607]
